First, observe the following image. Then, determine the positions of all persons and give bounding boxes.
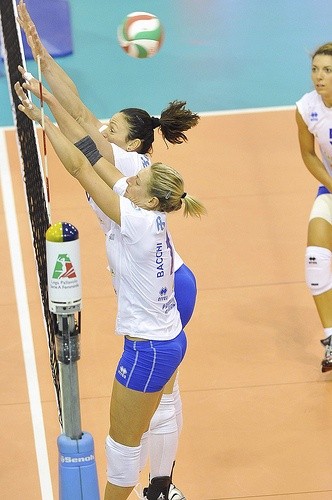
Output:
[293,41,332,377]
[14,0,188,500]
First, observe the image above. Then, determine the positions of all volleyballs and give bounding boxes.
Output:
[117,11,163,58]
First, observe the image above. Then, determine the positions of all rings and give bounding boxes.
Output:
[27,102,33,108]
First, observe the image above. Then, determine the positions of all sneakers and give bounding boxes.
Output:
[320,335,332,374]
[143,476,187,500]
